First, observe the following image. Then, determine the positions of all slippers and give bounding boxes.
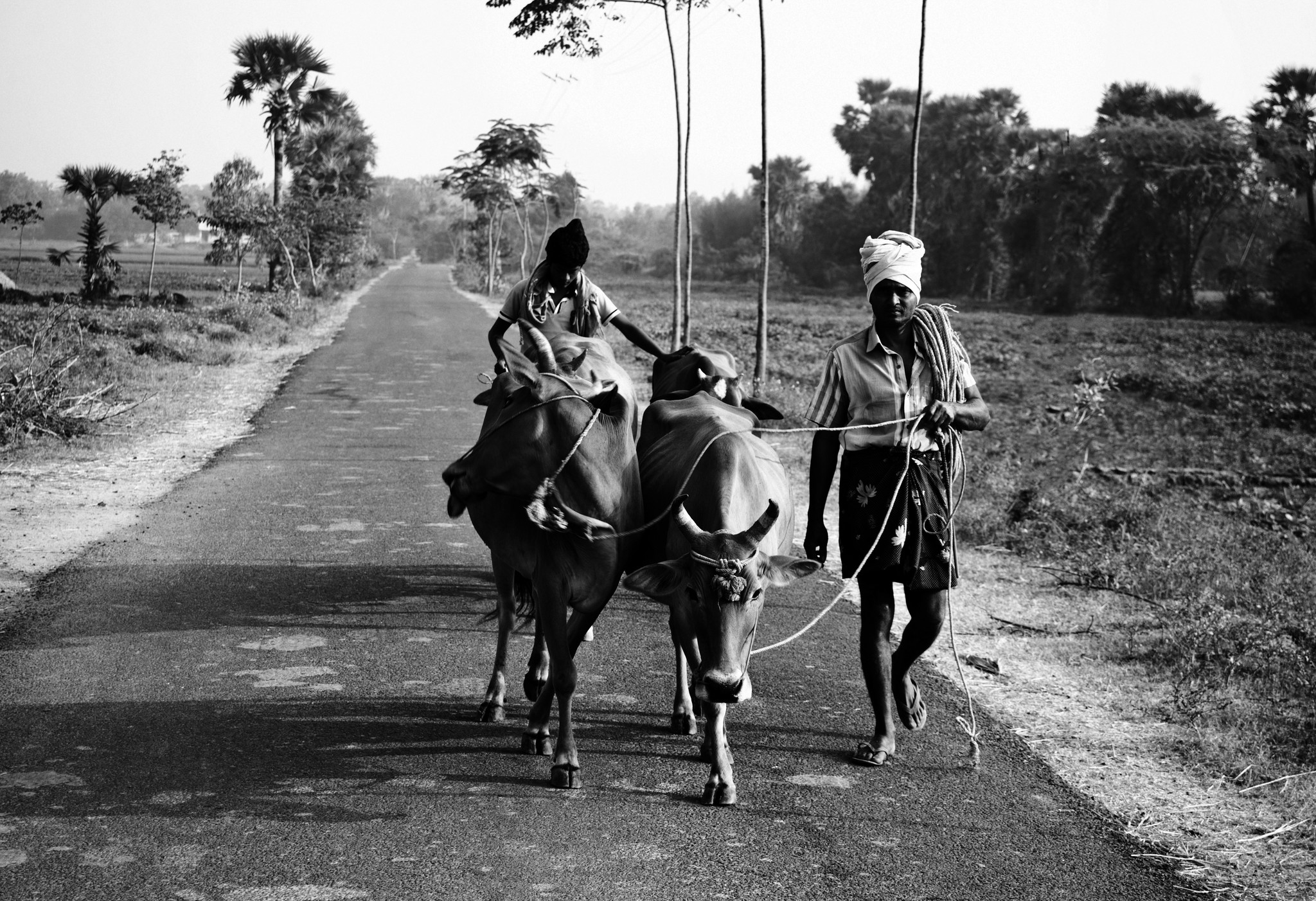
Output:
[851,742,891,766]
[891,677,928,732]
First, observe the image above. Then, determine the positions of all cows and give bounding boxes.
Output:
[649,342,786,420]
[622,389,823,808]
[441,318,646,790]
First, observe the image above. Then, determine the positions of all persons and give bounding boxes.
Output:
[802,229,990,765]
[487,218,669,377]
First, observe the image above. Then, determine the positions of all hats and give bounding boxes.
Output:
[545,219,590,262]
